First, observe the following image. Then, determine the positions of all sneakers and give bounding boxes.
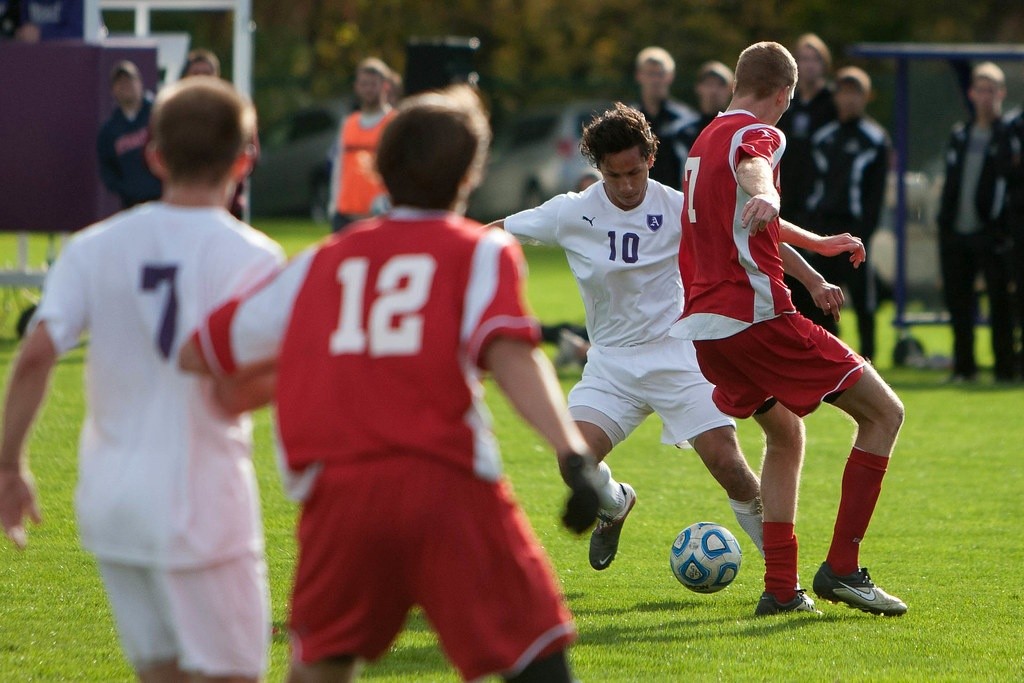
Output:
[813,561,908,615]
[588,482,637,570]
[754,589,813,616]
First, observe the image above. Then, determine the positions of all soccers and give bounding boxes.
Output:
[669,521,743,595]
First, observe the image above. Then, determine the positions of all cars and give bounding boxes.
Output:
[467,101,613,220]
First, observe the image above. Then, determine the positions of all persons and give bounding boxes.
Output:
[0,75,285,683]
[541,322,590,378]
[95,61,166,209]
[935,61,1024,388]
[326,57,402,234]
[803,69,891,366]
[660,59,735,193]
[178,47,245,224]
[776,34,837,314]
[667,42,909,616]
[629,46,701,177]
[15,23,39,41]
[480,102,845,605]
[180,85,600,683]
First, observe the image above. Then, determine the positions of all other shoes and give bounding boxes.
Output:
[946,370,975,387]
[994,372,1017,388]
[563,496,595,534]
